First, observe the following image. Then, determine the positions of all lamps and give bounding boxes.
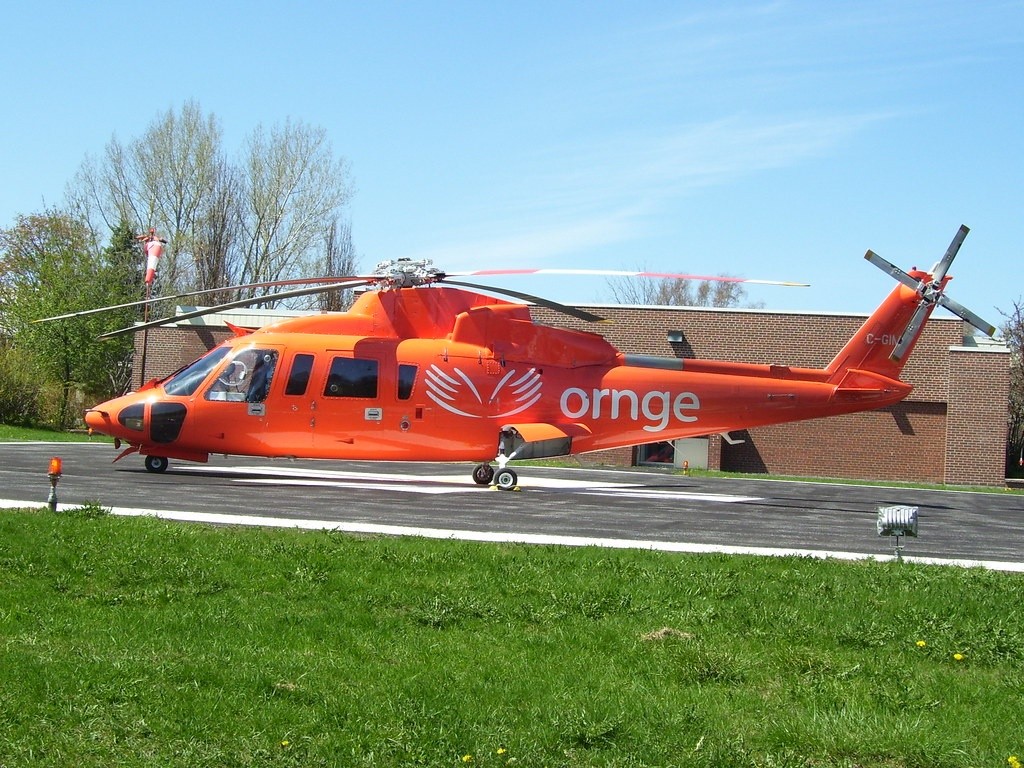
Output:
[877,504,919,539]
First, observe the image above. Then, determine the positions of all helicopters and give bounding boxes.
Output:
[29,223,998,493]
